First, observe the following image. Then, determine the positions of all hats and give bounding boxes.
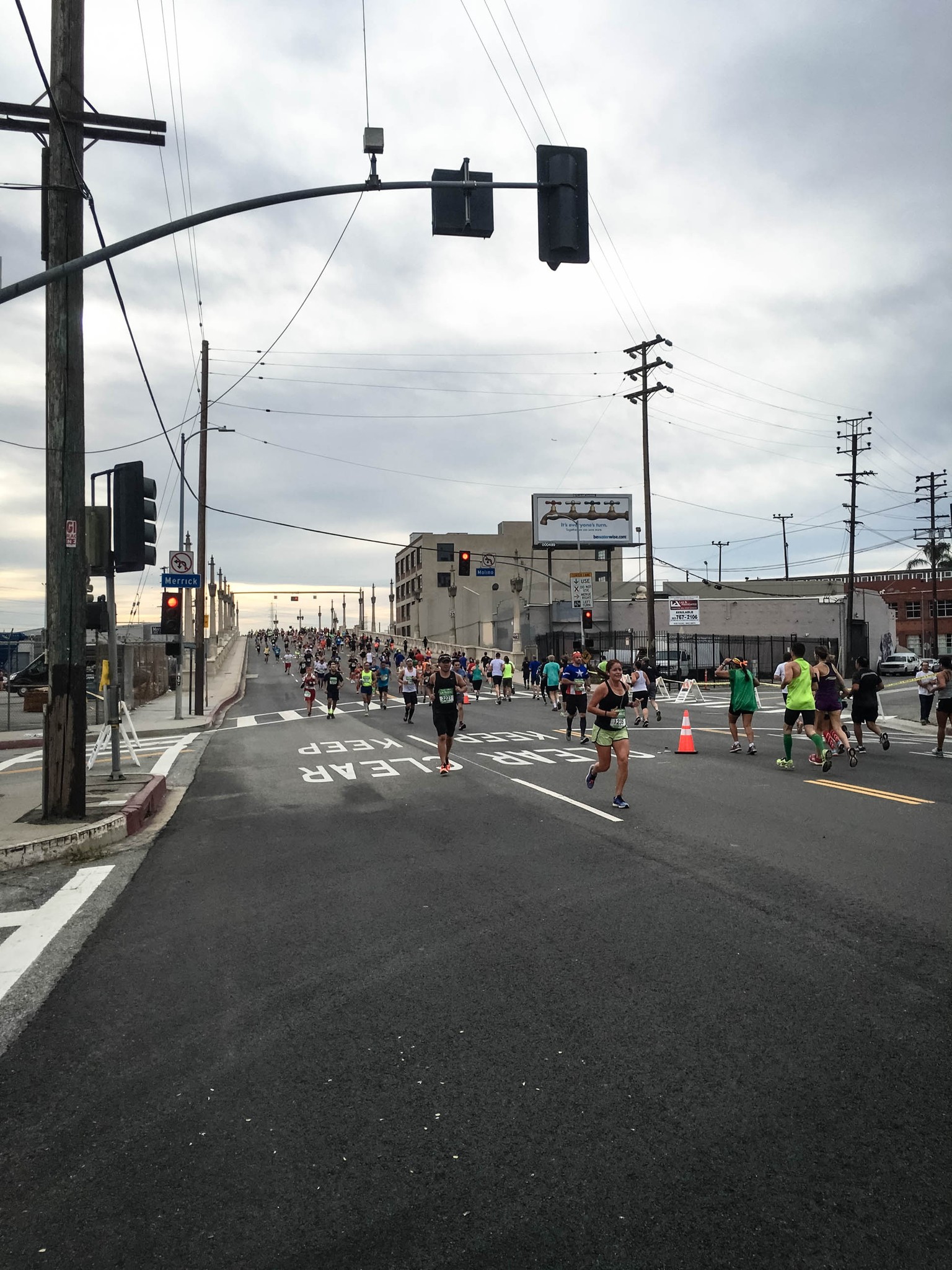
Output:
[439,655,451,662]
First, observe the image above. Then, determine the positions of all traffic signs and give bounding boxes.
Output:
[570,572,594,609]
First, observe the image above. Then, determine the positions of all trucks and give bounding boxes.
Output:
[647,634,721,682]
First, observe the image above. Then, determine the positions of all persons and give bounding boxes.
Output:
[916,661,937,725]
[641,657,661,722]
[397,658,418,724]
[451,659,468,730]
[715,656,760,754]
[774,652,803,734]
[562,651,591,744]
[630,661,650,727]
[426,654,469,775]
[585,659,644,808]
[776,642,832,772]
[847,656,890,753]
[596,656,608,683]
[808,645,857,768]
[248,626,578,719]
[823,654,850,756]
[929,656,952,757]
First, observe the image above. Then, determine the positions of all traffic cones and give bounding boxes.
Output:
[673,710,699,755]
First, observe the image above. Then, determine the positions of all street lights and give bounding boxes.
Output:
[172,424,237,721]
[635,527,641,585]
[704,561,708,582]
[547,513,586,656]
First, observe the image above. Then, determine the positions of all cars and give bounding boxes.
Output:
[879,652,922,677]
[921,658,942,673]
[2,657,181,702]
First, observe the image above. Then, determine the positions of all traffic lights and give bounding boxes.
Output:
[160,592,182,634]
[111,460,156,574]
[583,609,592,629]
[458,550,470,576]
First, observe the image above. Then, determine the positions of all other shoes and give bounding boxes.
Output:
[634,716,641,726]
[643,721,649,727]
[841,724,851,738]
[656,710,661,722]
[640,717,644,722]
[458,722,466,730]
[837,740,845,754]
[832,749,839,756]
[921,719,930,726]
[796,721,804,734]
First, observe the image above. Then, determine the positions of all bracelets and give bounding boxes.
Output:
[721,663,726,666]
[604,711,607,717]
[630,702,634,709]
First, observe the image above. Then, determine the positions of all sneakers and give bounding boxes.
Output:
[931,747,943,757]
[846,747,858,767]
[821,749,832,773]
[565,728,572,741]
[854,745,867,753]
[878,732,890,751]
[579,736,589,744]
[612,793,630,809]
[776,757,795,768]
[440,762,449,775]
[585,764,598,789]
[729,743,742,753]
[808,753,822,765]
[745,745,757,755]
[445,757,450,771]
